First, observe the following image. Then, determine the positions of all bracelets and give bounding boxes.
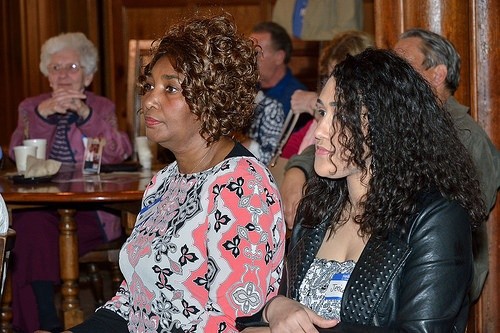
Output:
[265,295,284,324]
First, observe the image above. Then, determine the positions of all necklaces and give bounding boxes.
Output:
[189,149,213,175]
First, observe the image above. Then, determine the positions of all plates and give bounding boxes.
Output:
[101,163,141,172]
[12,173,57,184]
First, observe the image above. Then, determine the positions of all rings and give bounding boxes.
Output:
[70,99,76,105]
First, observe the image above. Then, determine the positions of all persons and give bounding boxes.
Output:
[8,33,133,333]
[35,8,500,333]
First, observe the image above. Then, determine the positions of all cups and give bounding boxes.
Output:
[136,137,153,168]
[23,139,47,160]
[13,145,38,175]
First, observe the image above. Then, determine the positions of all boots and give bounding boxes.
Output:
[33,280,61,331]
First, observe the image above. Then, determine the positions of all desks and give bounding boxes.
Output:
[0,174,157,333]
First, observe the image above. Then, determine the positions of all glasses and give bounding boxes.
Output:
[47,63,81,74]
